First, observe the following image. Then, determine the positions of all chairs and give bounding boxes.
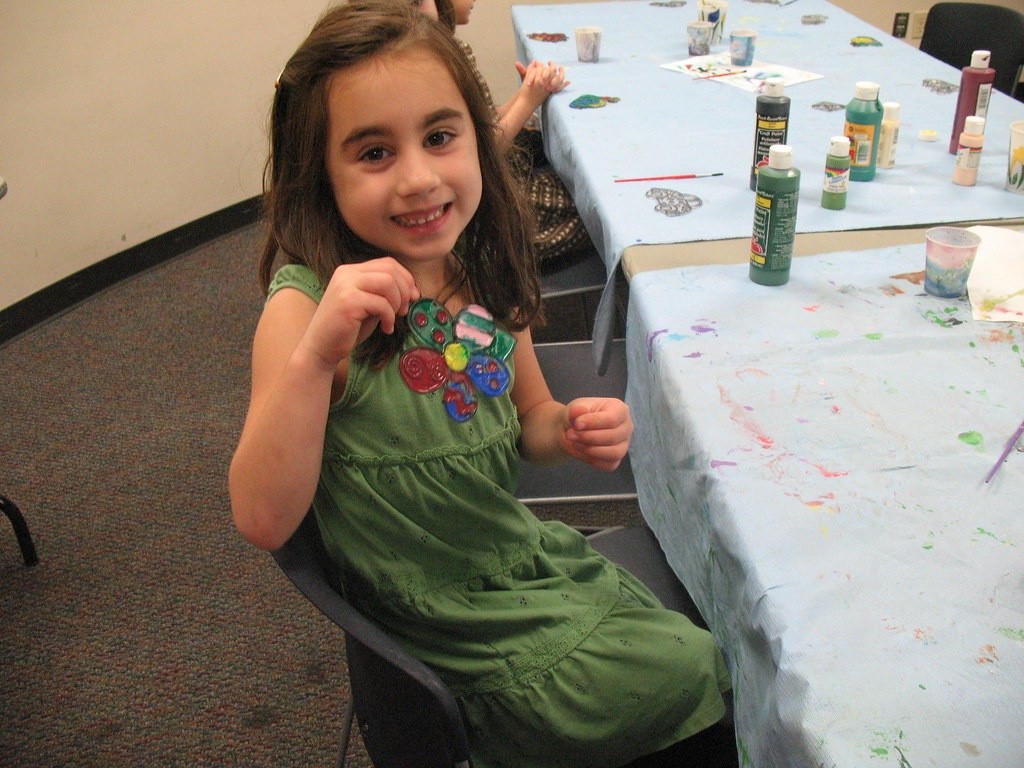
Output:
[271,340,713,768]
[919,2,1024,97]
[529,256,631,339]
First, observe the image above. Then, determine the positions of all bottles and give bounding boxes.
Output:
[952,116,985,186]
[748,144,800,286]
[842,82,883,182]
[875,101,900,168]
[750,77,790,191]
[822,137,851,211]
[949,50,995,155]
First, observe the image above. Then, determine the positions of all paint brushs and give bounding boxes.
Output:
[614,173,724,183]
[694,70,747,80]
[986,421,1024,483]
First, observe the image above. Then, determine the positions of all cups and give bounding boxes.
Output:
[687,21,716,56]
[923,226,982,299]
[575,27,603,63]
[697,0,729,46]
[1006,121,1024,194]
[729,29,759,66]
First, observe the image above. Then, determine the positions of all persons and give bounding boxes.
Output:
[231,0,734,768]
[412,0,598,279]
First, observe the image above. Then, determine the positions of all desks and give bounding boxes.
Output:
[514,1,1024,766]
